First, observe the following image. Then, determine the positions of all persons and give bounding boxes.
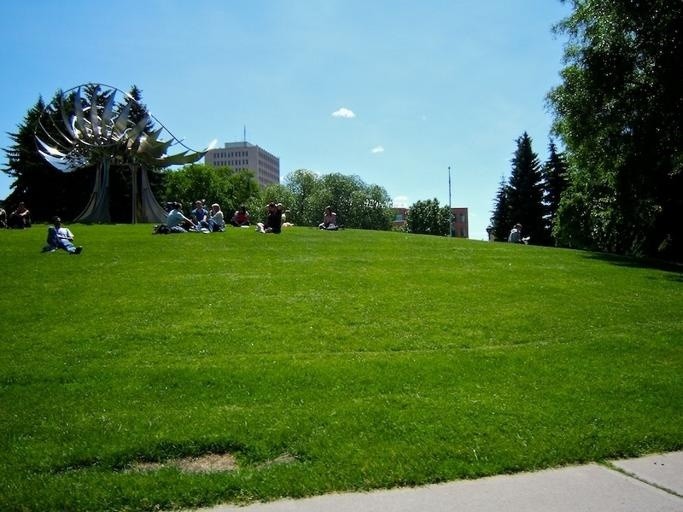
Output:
[0,200,9,230]
[47,216,83,254]
[160,199,226,234]
[8,201,32,230]
[508,223,524,243]
[318,205,339,232]
[262,201,292,234]
[230,205,253,228]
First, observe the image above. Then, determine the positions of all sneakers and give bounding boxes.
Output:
[50,246,83,254]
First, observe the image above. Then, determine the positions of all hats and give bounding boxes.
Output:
[267,202,275,207]
